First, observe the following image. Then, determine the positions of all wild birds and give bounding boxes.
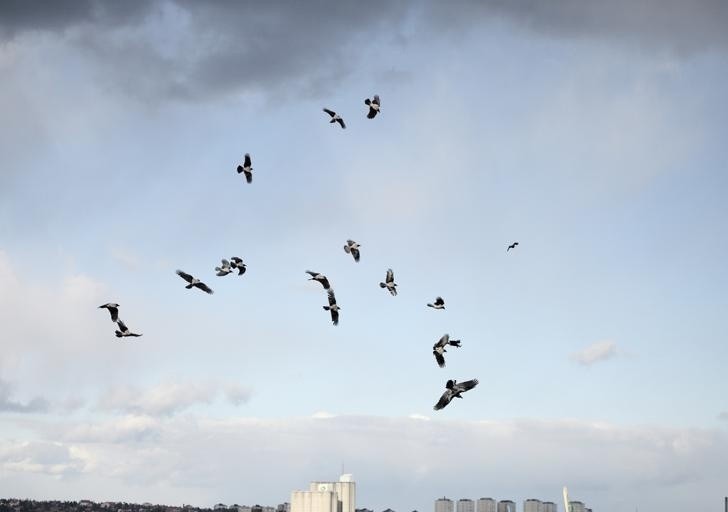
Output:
[233,153,254,183]
[364,94,385,119]
[174,257,246,295]
[98,302,142,339]
[322,107,346,131]
[426,296,446,310]
[305,270,341,326]
[379,268,398,297]
[432,333,461,370]
[506,241,519,250]
[432,377,480,410]
[340,238,363,263]
